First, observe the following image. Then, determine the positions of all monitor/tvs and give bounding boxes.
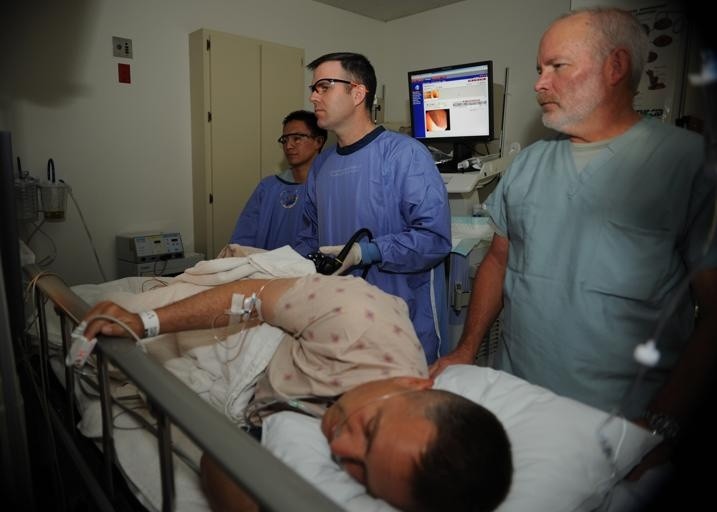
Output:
[407,60,494,174]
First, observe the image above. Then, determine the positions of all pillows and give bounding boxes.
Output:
[259,363,665,512]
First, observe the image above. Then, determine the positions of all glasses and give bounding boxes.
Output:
[277,133,320,146]
[308,78,369,97]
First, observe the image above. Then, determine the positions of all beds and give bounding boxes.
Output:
[20,237,686,512]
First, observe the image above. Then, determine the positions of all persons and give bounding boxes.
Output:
[422,6,716,471]
[78,274,512,512]
[229,109,328,252]
[292,50,452,369]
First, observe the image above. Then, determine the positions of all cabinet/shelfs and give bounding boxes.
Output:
[189,28,305,261]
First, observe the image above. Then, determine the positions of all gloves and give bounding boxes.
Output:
[318,242,361,277]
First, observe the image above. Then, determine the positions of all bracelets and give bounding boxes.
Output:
[136,310,161,340]
